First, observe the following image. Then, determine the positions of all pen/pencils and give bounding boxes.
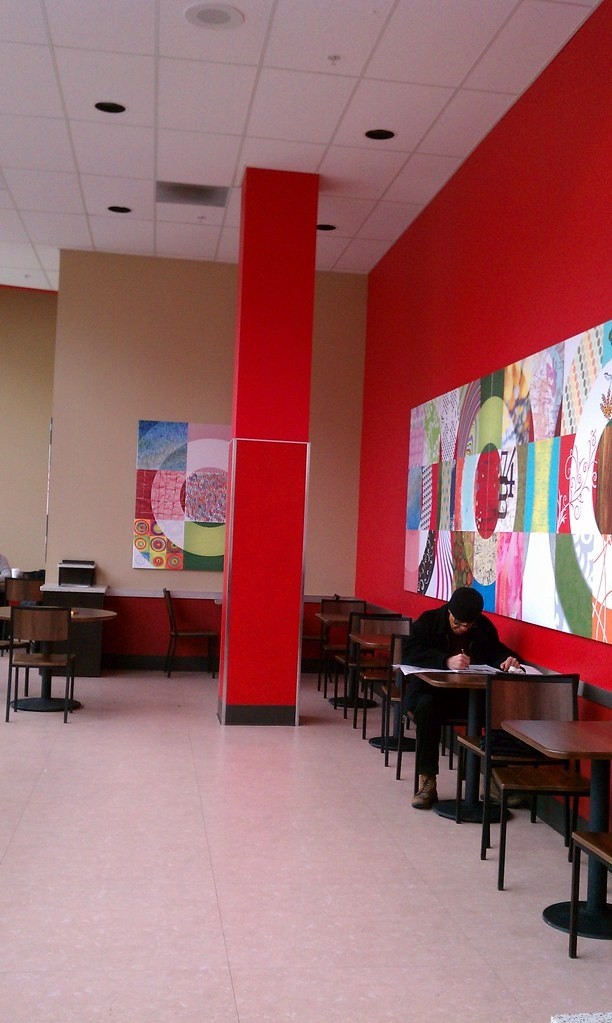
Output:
[462,648,469,669]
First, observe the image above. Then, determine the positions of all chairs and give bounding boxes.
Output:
[302,594,612,961]
[162,587,218,679]
[0,570,77,724]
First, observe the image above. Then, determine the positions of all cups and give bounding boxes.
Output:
[12,568,19,578]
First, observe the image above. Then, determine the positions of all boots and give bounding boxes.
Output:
[412,770,438,808]
[482,773,523,806]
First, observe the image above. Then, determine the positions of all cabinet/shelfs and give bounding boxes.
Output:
[39,583,106,678]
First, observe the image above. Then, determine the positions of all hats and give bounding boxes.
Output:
[447,587,484,622]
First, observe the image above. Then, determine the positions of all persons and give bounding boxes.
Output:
[401,586,525,810]
[0,555,11,582]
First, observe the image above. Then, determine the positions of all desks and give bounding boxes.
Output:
[214,598,367,606]
[347,634,416,752]
[501,720,612,943]
[315,613,378,709]
[413,664,514,823]
[0,606,117,712]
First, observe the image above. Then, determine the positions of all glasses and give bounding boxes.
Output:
[454,618,471,626]
